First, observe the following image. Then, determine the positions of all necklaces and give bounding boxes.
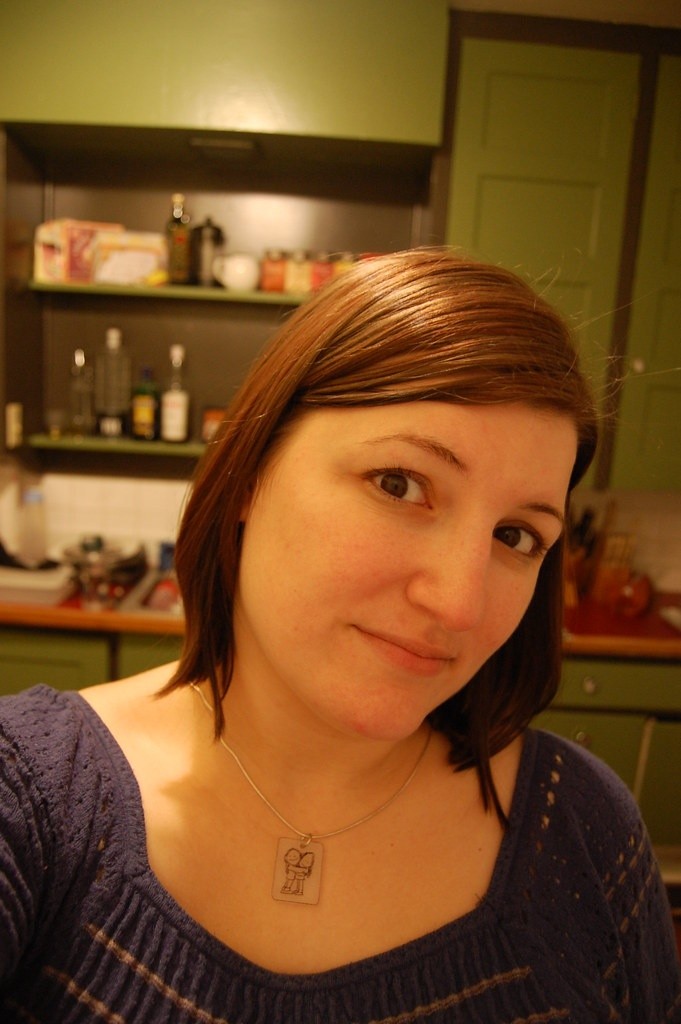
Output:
[189,682,434,905]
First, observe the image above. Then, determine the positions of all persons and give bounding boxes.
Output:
[0,250,681,1024]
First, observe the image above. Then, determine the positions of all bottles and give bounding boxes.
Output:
[65,328,193,444]
[159,190,223,286]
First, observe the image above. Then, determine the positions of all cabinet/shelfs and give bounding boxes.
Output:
[1,1,681,494]
[0,621,681,890]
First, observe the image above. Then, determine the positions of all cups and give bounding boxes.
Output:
[211,254,258,292]
